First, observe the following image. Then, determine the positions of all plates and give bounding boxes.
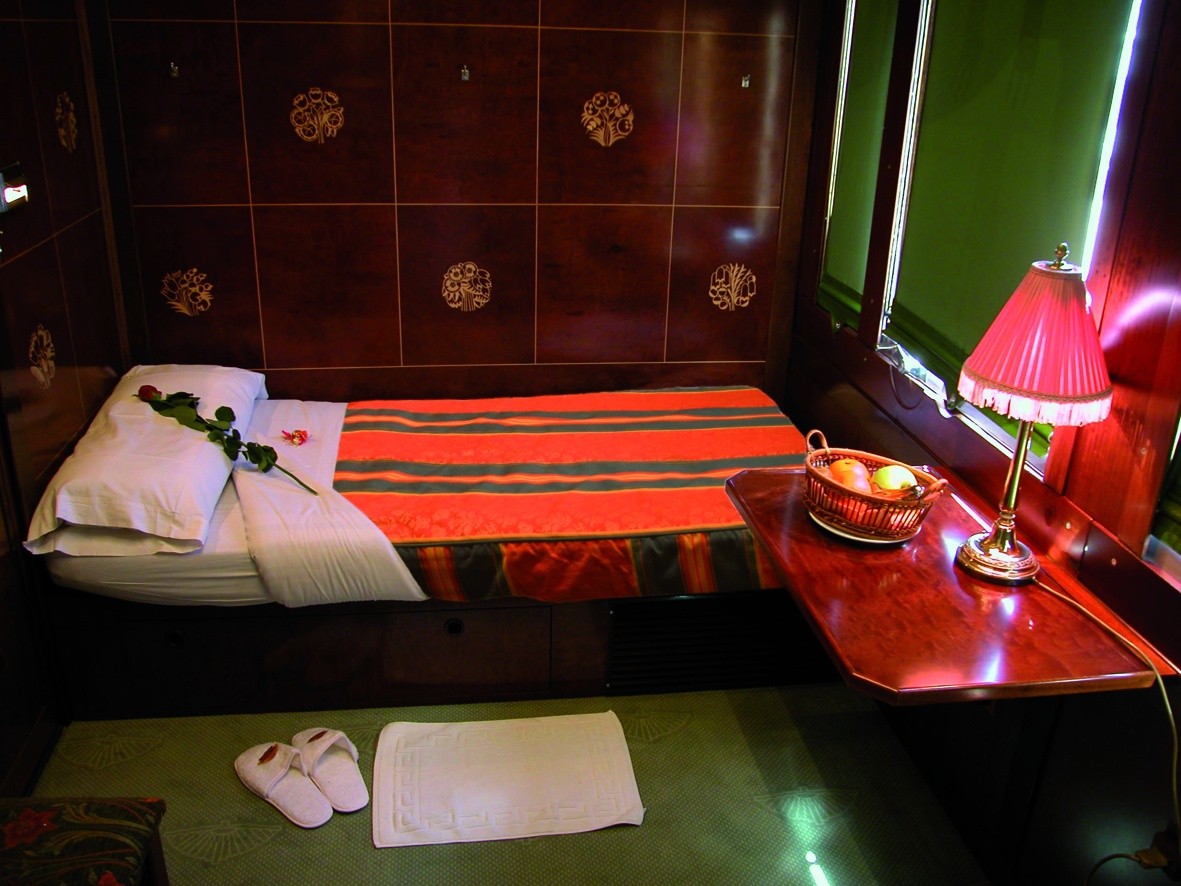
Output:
[807,512,921,543]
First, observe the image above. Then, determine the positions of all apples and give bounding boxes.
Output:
[815,459,919,512]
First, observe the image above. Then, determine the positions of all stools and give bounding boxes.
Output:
[1,796,171,886]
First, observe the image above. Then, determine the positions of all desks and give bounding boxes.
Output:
[724,465,1181,707]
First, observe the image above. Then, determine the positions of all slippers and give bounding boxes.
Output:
[235,727,369,828]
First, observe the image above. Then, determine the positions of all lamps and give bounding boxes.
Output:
[958,242,1114,586]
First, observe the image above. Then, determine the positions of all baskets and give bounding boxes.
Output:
[803,429,948,542]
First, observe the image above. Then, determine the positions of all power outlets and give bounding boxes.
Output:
[1154,821,1181,886]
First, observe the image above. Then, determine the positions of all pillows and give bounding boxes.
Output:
[23,365,270,555]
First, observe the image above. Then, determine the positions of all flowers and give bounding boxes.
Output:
[132,384,320,498]
[279,428,310,446]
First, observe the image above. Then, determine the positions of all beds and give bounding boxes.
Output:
[47,384,822,723]
